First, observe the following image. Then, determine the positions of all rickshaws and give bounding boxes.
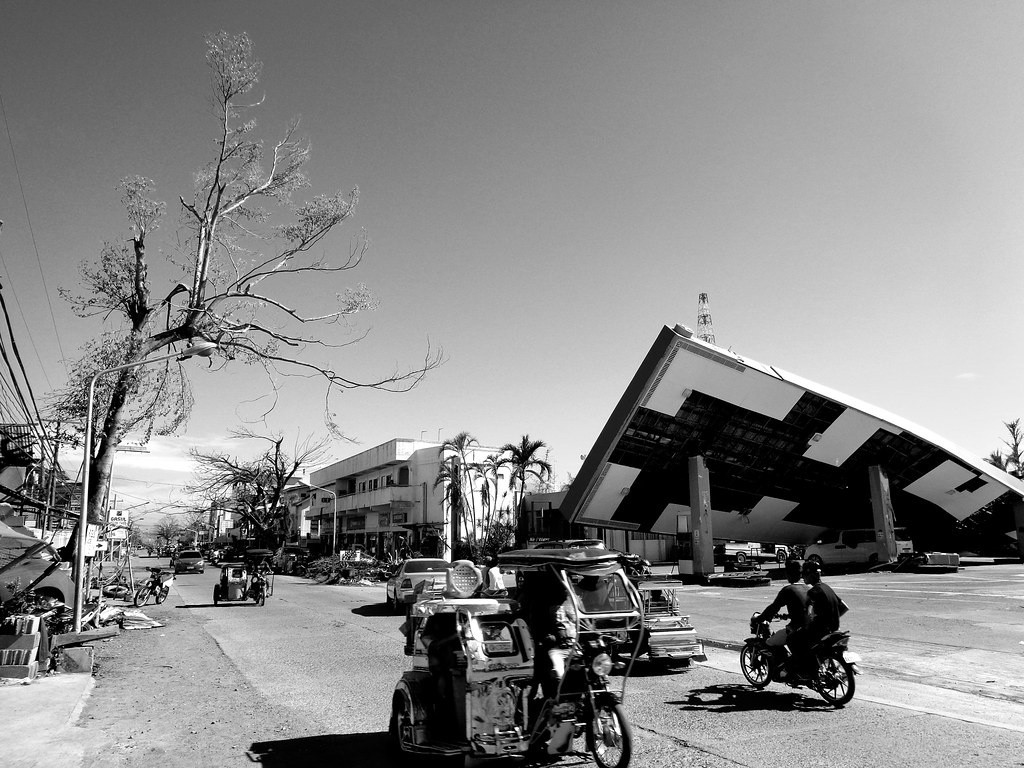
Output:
[386,547,646,768]
[213,563,274,606]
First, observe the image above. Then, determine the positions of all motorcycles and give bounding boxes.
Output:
[155,548,162,559]
[146,548,154,557]
[134,566,176,608]
[497,537,649,670]
[169,552,179,568]
[473,582,508,598]
[210,552,219,566]
[740,611,864,705]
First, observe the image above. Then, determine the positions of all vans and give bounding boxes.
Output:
[804,527,914,569]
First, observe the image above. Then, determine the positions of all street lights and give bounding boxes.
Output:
[199,523,215,542]
[298,480,336,554]
[187,529,198,551]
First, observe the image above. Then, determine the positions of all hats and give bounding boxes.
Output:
[800,561,822,572]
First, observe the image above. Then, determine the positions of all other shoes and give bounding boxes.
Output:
[793,673,812,681]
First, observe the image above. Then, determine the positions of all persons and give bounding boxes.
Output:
[786,562,839,678]
[157,546,160,559]
[148,547,151,557]
[481,556,507,597]
[751,562,812,670]
[646,590,666,601]
[251,555,272,597]
[534,574,613,696]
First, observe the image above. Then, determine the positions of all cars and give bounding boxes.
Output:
[153,545,176,556]
[347,552,385,568]
[113,544,144,557]
[172,550,209,574]
[386,558,451,608]
[0,535,85,615]
